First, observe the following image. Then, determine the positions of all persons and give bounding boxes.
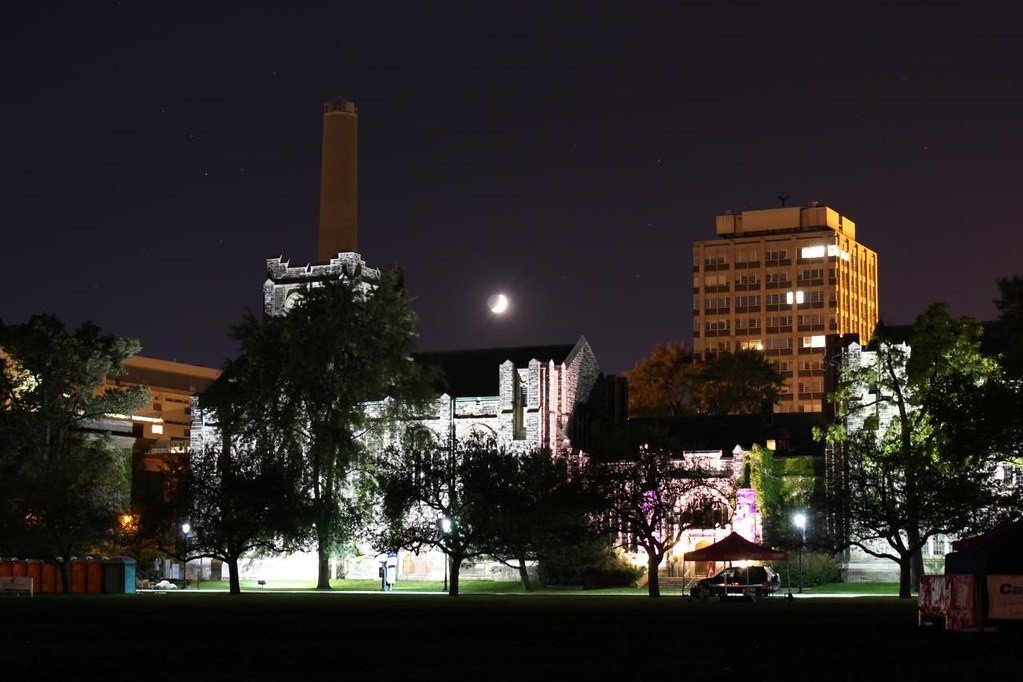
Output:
[381,562,393,591]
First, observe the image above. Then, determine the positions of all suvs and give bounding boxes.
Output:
[697,565,782,596]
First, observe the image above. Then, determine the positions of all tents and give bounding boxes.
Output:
[682,532,795,600]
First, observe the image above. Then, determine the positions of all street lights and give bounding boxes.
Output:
[794,514,806,592]
[182,523,191,588]
[441,519,452,590]
